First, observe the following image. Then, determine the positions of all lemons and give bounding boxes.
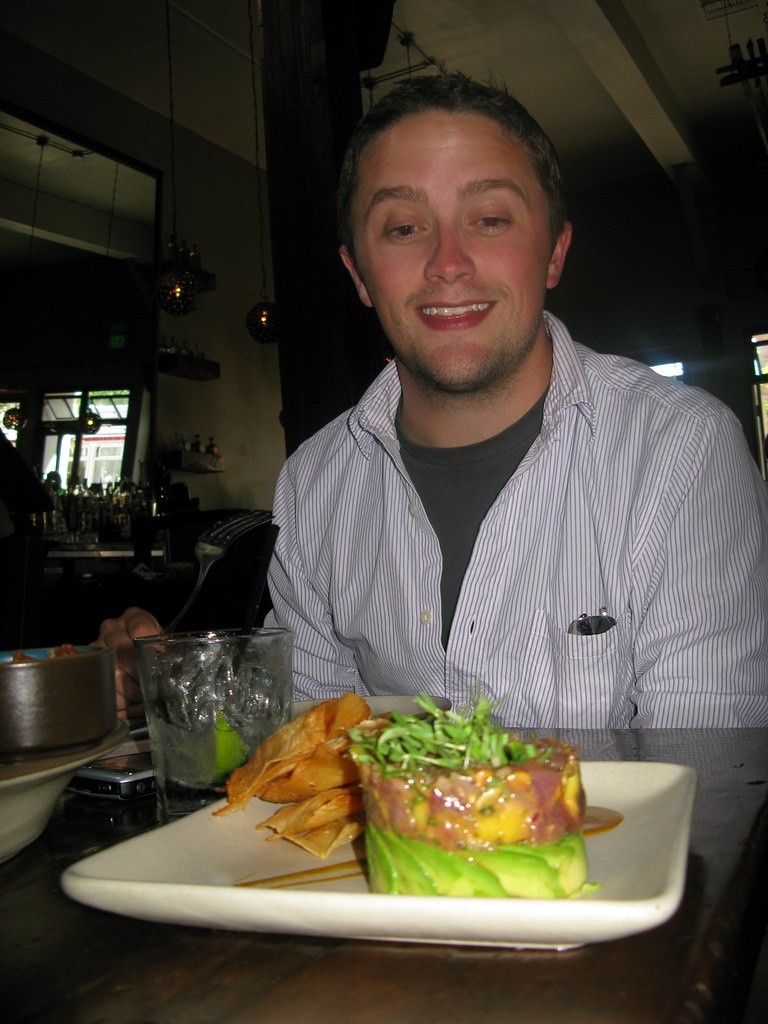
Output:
[167,710,246,785]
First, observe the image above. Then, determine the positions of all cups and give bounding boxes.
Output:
[134,627,294,822]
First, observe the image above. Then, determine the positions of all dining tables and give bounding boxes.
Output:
[0,718,768,1024]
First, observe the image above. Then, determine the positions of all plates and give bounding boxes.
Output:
[60,763,697,951]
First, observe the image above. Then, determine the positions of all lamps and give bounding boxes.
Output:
[77,412,104,435]
[151,0,205,315]
[245,0,280,347]
[3,407,29,432]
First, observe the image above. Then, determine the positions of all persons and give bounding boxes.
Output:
[93,69,768,728]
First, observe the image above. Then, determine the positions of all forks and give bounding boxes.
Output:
[159,509,276,633]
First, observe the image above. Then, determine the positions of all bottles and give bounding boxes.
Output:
[179,432,219,456]
[160,336,200,358]
[31,478,131,541]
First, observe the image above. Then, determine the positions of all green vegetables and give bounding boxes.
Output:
[347,692,552,765]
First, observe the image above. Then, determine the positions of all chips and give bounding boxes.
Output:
[213,687,372,856]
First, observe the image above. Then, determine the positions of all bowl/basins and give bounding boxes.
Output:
[0,644,116,763]
[0,718,130,863]
[292,694,451,742]
